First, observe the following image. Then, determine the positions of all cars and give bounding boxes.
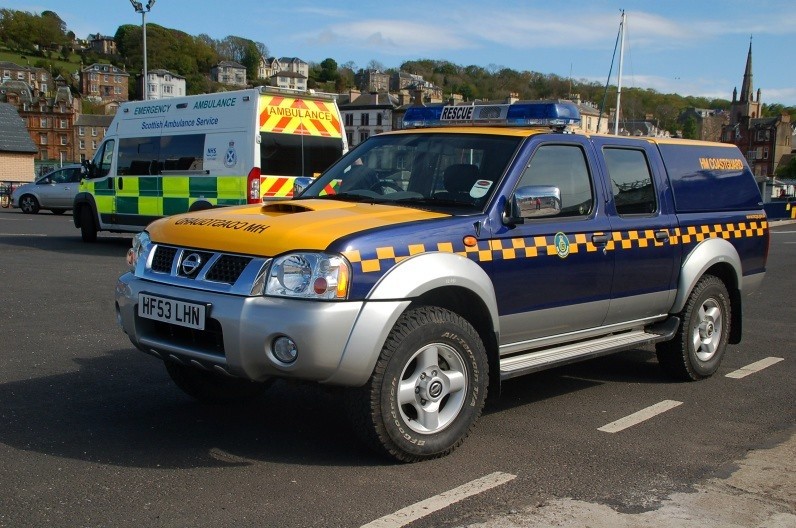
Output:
[10,162,111,216]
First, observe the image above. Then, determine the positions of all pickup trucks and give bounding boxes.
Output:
[113,99,771,463]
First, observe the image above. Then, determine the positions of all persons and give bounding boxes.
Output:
[516,195,557,212]
[36,158,91,182]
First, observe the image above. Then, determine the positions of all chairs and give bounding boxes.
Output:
[433,164,486,209]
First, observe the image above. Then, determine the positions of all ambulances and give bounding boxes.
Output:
[67,84,351,242]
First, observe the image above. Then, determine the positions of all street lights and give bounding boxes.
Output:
[130,0,155,101]
